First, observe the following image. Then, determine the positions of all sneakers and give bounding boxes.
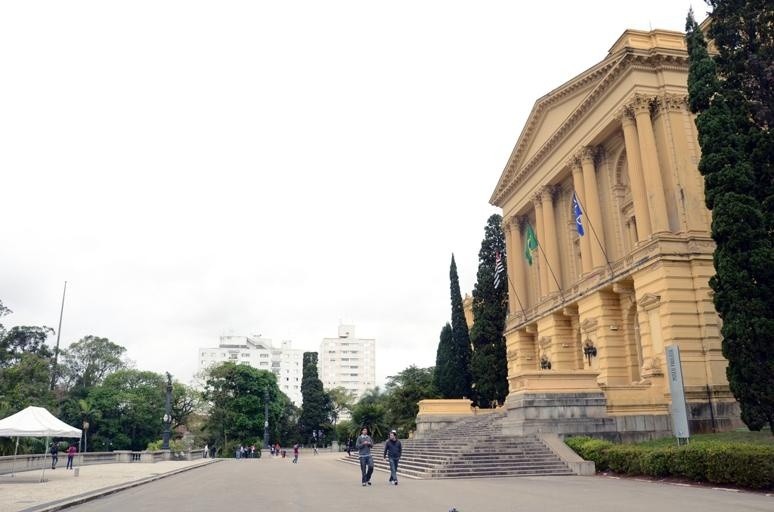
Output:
[362,478,398,486]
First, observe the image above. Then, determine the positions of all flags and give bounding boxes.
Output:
[523,223,539,267]
[493,250,506,290]
[571,193,585,237]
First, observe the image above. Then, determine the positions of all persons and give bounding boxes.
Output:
[356,426,375,487]
[344,437,353,456]
[64,442,77,470]
[49,440,59,470]
[383,430,402,485]
[201,440,320,464]
